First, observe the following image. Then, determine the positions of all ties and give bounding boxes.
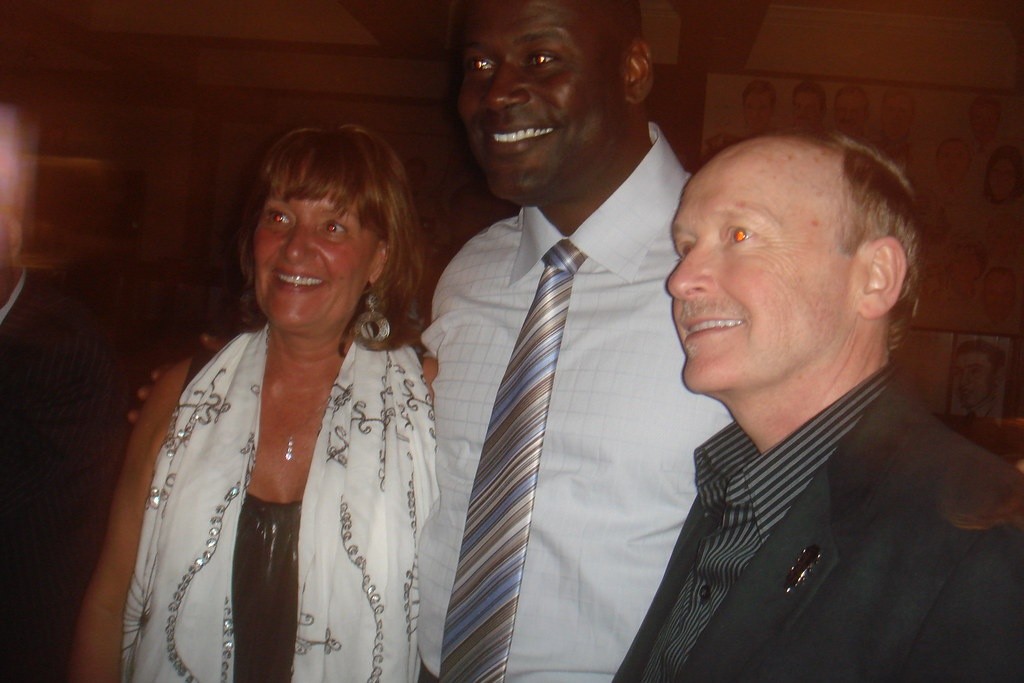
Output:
[438,239,587,683]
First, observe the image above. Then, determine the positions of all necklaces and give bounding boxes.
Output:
[278,412,315,461]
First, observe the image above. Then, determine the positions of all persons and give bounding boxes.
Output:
[613,121,1023,683]
[68,121,442,683]
[128,0,734,682]
[0,106,118,683]
